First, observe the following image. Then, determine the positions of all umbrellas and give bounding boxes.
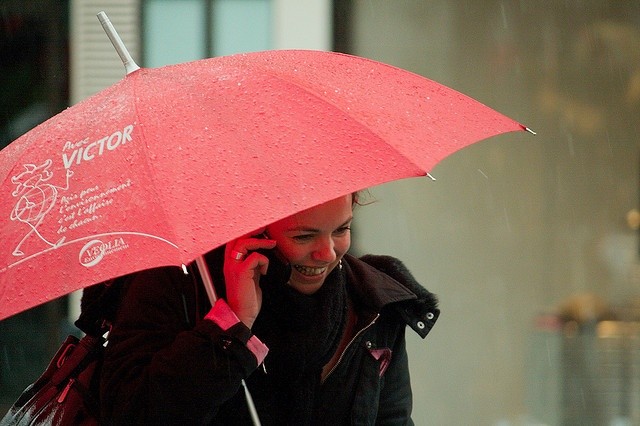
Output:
[0,11,537,425]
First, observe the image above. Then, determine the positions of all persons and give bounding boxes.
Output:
[97,184,441,426]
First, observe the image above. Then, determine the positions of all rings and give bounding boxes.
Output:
[230,250,245,261]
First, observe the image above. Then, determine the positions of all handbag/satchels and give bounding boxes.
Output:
[1,334,100,426]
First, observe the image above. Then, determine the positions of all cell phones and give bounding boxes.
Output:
[243,233,292,286]
